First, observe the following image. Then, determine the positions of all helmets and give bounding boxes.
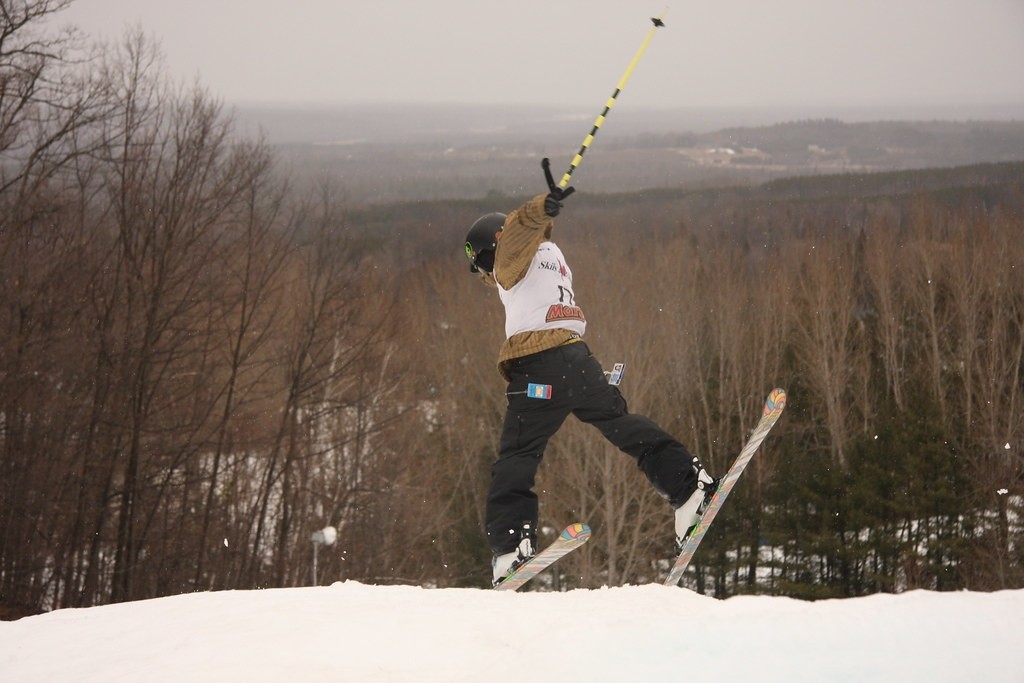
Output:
[464,212,507,273]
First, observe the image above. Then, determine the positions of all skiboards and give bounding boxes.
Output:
[492,387,787,590]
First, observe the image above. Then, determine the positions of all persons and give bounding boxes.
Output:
[464,187,720,587]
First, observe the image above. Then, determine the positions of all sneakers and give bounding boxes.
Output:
[491,520,535,588]
[674,456,722,558]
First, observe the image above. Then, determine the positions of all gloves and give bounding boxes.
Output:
[545,197,564,217]
